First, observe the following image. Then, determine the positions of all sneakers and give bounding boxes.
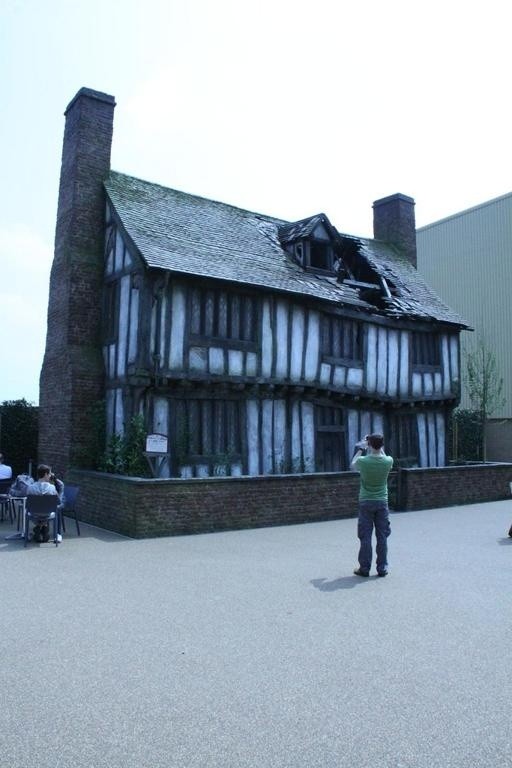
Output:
[55,534,62,542]
[33,526,50,542]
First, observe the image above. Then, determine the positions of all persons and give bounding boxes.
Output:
[0,453,67,543]
[350,432,394,577]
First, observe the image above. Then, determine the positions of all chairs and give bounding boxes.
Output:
[0,482,80,548]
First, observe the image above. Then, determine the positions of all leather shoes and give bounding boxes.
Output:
[353,567,370,576]
[378,570,389,577]
